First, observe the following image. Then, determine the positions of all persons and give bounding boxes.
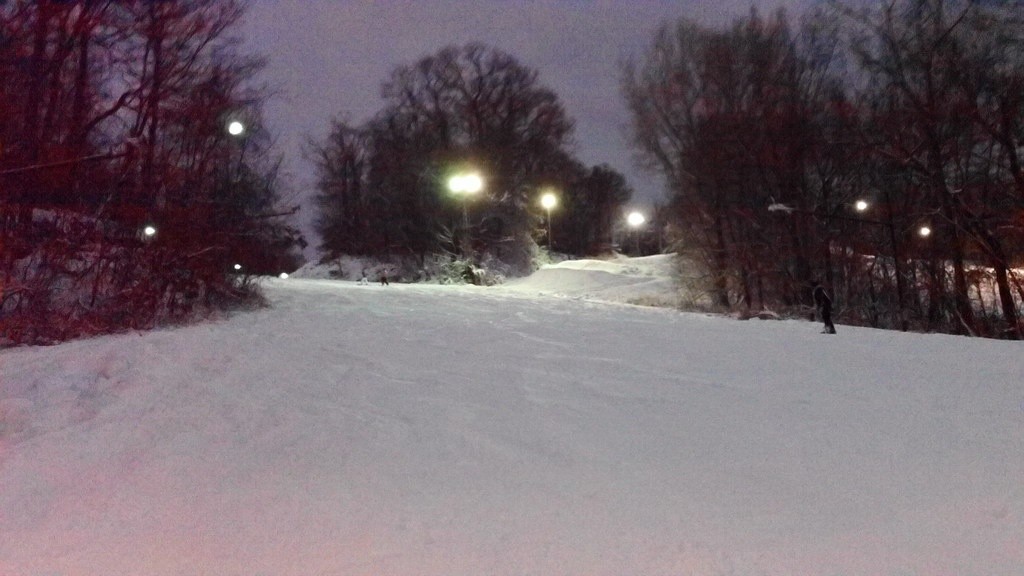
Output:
[361,267,369,285]
[382,268,389,286]
[810,278,837,334]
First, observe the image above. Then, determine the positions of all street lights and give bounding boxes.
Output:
[452,177,477,257]
[855,185,905,330]
[544,195,554,254]
[631,214,641,256]
